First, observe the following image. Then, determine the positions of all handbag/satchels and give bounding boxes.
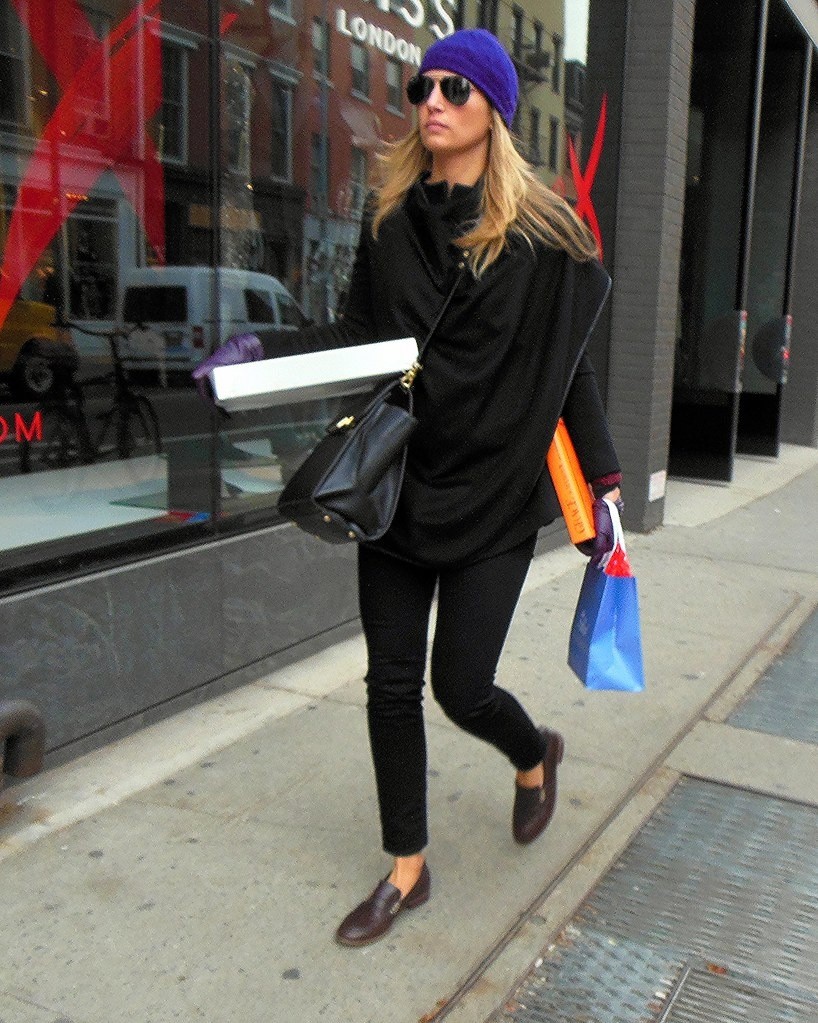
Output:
[278,382,416,546]
[566,497,645,692]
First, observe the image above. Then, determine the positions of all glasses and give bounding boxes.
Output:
[406,75,475,107]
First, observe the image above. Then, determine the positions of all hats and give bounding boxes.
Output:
[418,28,518,130]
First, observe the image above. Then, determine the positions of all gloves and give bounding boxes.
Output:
[575,499,614,557]
[191,331,263,419]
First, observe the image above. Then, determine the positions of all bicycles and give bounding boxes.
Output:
[19,320,167,477]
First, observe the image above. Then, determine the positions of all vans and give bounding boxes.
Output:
[66,263,317,390]
[0,212,80,403]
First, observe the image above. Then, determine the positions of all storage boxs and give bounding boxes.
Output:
[209,338,418,415]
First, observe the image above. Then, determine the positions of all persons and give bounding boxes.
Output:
[190,29,624,948]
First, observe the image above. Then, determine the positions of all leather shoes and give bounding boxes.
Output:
[512,730,563,845]
[334,861,430,944]
